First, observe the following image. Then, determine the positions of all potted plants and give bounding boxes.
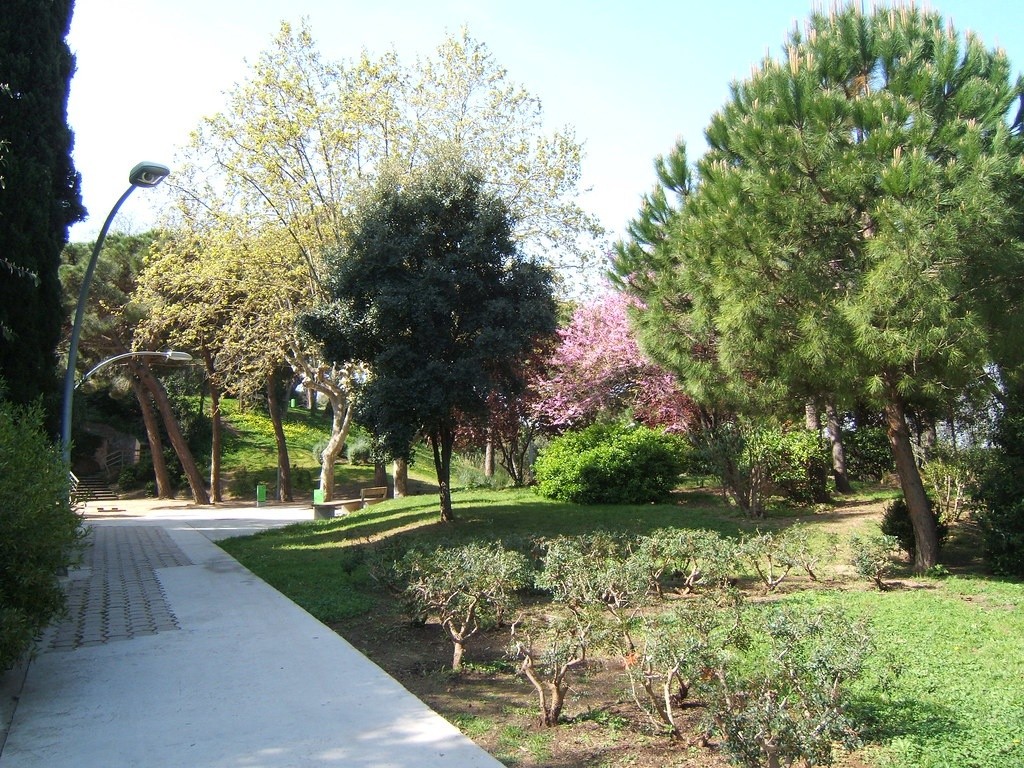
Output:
[115,488,127,500]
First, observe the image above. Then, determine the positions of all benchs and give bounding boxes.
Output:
[311,498,378,520]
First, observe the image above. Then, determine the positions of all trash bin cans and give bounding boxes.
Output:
[314,489,324,503]
[257,484,266,502]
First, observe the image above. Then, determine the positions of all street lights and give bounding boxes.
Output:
[59,160,193,517]
